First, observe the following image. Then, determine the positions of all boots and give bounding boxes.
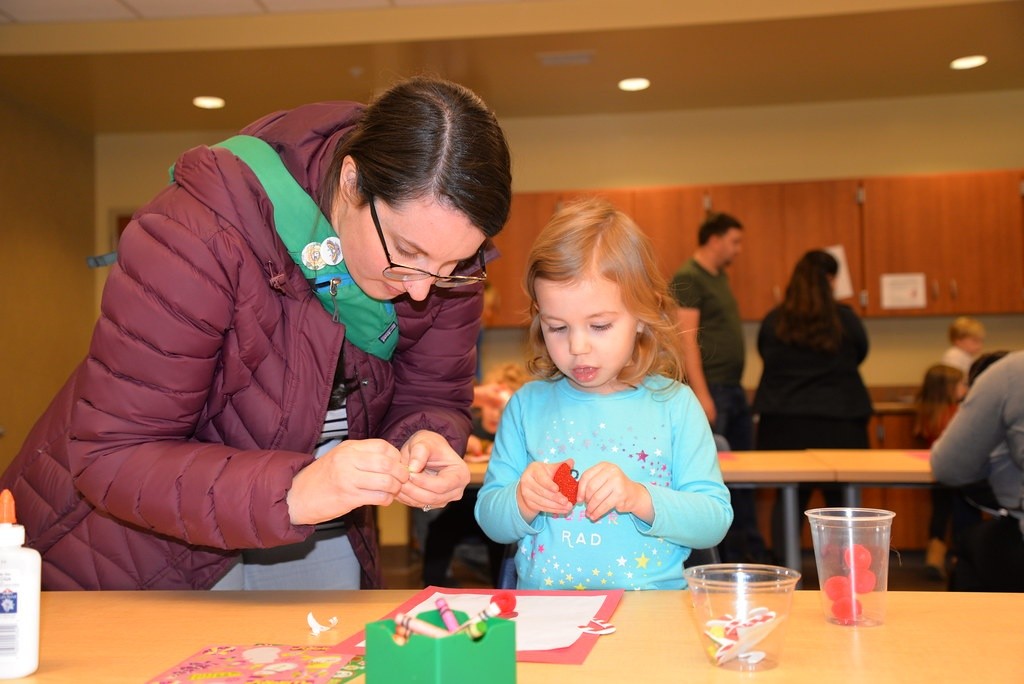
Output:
[925,538,947,581]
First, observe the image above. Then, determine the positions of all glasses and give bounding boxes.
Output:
[359,169,488,288]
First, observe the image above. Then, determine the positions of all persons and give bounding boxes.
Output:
[912,317,1024,592]
[663,213,767,570]
[751,250,875,566]
[474,200,736,592]
[421,382,520,590]
[0,75,514,592]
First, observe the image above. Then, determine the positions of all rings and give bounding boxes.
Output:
[422,504,432,512]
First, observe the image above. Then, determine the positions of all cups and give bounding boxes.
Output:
[683,563,801,671]
[804,507,897,628]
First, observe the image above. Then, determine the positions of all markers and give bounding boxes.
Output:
[394,596,501,643]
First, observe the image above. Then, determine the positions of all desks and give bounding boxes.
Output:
[467,448,936,589]
[0,589,1024,684]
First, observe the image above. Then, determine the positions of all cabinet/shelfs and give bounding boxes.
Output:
[864,169,1024,319]
[486,184,707,330]
[709,178,862,323]
